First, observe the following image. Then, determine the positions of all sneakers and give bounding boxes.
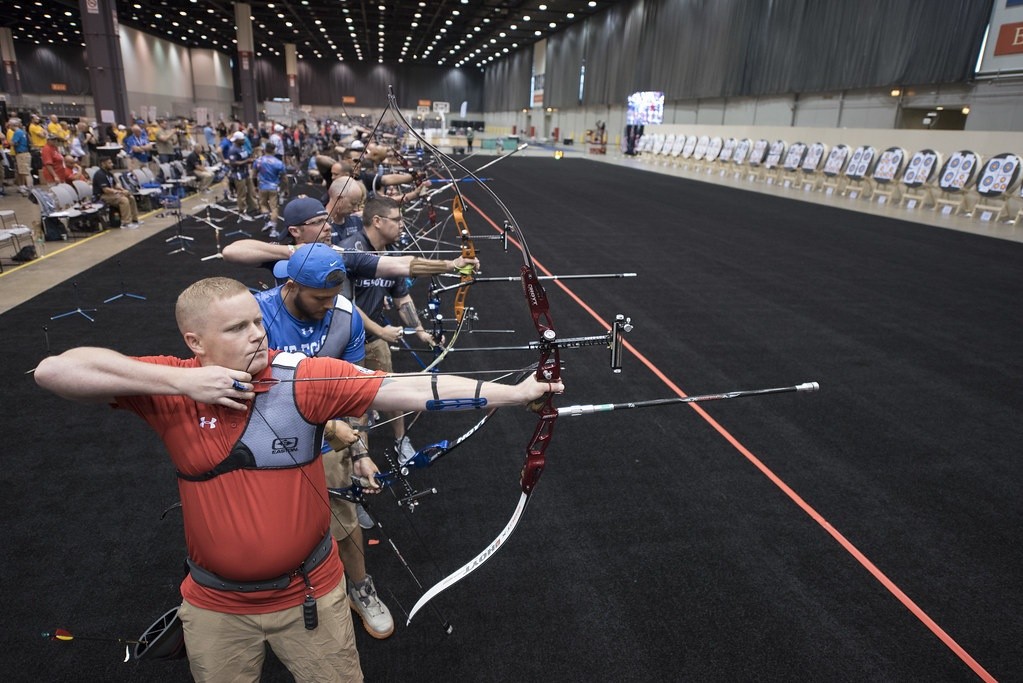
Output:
[347,574,394,639]
[394,435,417,466]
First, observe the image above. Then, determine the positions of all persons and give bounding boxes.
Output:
[186,143,215,193]
[91,157,143,229]
[251,143,286,238]
[496,137,504,151]
[466,127,473,153]
[0,110,564,683]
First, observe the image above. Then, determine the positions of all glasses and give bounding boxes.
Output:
[372,216,401,222]
[287,217,334,233]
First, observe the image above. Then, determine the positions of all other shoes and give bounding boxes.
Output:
[372,410,380,420]
[261,222,279,237]
[120,221,144,229]
[356,503,374,529]
[16,184,32,197]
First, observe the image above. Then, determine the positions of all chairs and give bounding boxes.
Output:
[0,145,228,272]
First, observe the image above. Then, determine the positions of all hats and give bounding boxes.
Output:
[31,114,40,119]
[273,243,346,289]
[230,131,245,142]
[349,140,368,154]
[274,124,284,132]
[8,120,19,126]
[47,133,58,140]
[278,195,328,241]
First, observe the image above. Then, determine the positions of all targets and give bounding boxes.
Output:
[636,135,806,170]
[903,152,937,186]
[977,152,1019,194]
[873,151,903,179]
[802,146,825,170]
[941,151,976,189]
[846,149,873,176]
[822,146,847,174]
